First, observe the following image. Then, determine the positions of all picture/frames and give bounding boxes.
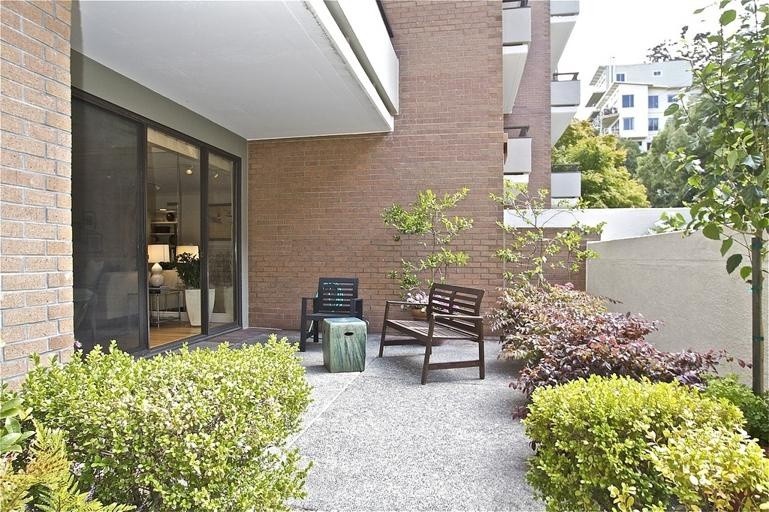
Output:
[127,289,181,329]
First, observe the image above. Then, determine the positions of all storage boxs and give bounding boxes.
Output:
[377,282,486,385]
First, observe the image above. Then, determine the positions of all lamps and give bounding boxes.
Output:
[298,276,364,353]
[73,257,108,347]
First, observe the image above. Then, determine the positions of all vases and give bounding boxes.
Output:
[381,187,474,316]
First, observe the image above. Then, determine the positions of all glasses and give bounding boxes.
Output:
[186,162,194,174]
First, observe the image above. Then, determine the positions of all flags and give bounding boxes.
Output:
[410,308,456,347]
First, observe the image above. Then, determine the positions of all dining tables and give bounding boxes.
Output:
[173,252,217,327]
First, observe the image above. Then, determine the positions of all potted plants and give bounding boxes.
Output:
[207,202,233,241]
